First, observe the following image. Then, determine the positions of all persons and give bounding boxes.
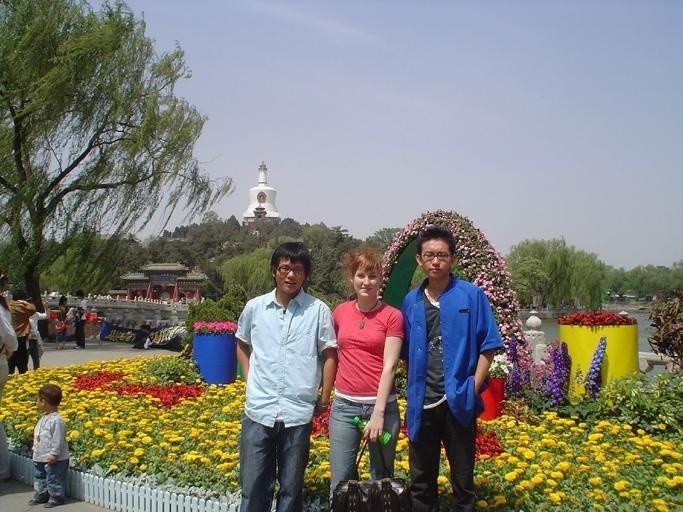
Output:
[234,239,336,511]
[394,226,501,511]
[131,323,151,350]
[6,287,90,376]
[28,383,70,508]
[320,242,404,512]
[0,272,18,481]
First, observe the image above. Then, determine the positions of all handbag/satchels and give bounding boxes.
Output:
[330,476,413,512]
[55,321,66,334]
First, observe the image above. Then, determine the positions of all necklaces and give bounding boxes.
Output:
[357,299,379,329]
[36,414,48,442]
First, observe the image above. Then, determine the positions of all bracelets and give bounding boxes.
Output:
[316,399,329,409]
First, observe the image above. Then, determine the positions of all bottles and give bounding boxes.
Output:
[352,416,391,445]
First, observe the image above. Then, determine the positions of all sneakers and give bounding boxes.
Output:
[29,497,45,506]
[44,501,59,508]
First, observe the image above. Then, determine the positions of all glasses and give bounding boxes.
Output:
[275,265,306,275]
[420,252,452,261]
